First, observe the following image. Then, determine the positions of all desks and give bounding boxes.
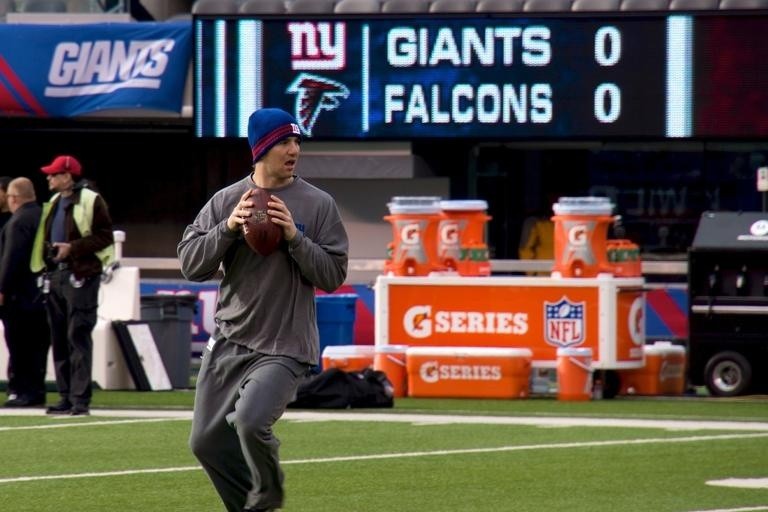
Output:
[371,273,654,400]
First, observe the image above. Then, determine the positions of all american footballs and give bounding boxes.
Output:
[242,189,283,255]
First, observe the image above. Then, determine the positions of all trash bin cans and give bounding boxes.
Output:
[140,293,199,390]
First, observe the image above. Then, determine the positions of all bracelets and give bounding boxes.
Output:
[289,229,304,249]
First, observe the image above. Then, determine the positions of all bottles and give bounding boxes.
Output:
[605,238,641,278]
[455,241,490,278]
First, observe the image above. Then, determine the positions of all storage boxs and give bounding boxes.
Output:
[320,344,376,373]
[615,341,688,397]
[404,344,534,400]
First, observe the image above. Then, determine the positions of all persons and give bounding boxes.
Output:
[28,155,114,416]
[0,177,18,226]
[175,108,348,512]
[517,189,564,275]
[0,176,51,407]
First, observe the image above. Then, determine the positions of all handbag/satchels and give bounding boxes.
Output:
[292,367,395,408]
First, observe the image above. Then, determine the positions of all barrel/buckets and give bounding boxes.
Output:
[372,345,408,399]
[556,345,595,402]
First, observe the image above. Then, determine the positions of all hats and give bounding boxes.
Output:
[41,154,81,175]
[247,107,303,164]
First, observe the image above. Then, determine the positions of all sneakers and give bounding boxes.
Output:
[71,404,88,415]
[46,400,71,414]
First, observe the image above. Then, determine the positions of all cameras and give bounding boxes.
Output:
[43,241,58,259]
